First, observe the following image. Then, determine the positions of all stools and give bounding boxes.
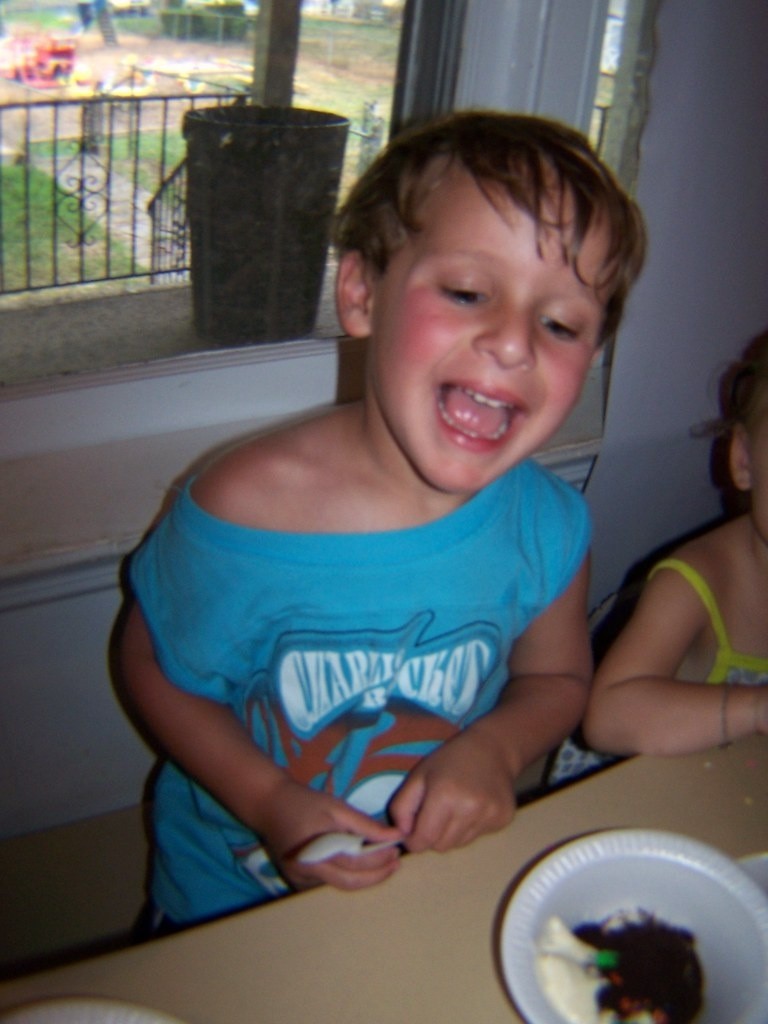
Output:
[0,804,147,970]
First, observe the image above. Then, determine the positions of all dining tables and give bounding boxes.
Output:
[0,730,768,1024]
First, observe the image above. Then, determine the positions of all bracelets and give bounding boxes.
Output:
[722,687,729,745]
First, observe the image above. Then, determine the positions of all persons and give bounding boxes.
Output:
[119,112,647,944]
[548,329,768,783]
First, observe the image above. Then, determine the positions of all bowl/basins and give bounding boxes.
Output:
[499,826,768,1024]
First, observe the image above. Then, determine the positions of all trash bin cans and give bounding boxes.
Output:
[185,105,349,339]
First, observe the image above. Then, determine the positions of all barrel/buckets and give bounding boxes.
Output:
[180,105,352,347]
